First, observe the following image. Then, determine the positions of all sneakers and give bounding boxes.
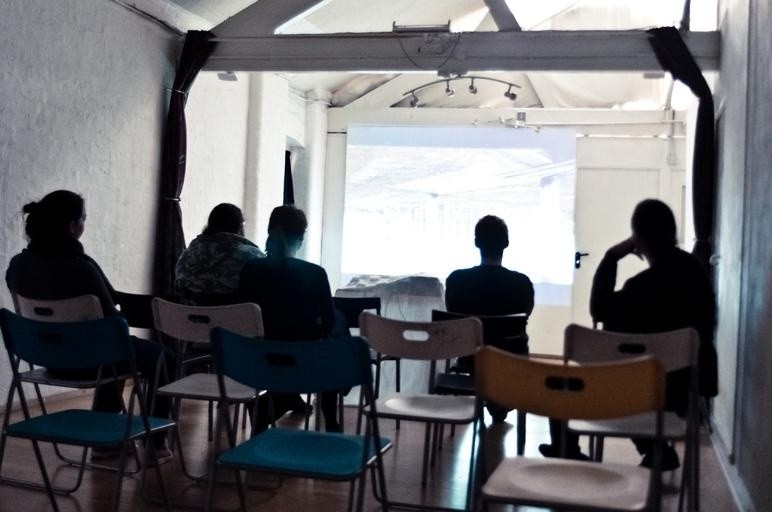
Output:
[144,444,173,465]
[288,396,314,415]
[90,443,139,459]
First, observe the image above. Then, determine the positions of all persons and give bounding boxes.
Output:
[444,212,535,424]
[538,197,722,476]
[239,205,346,438]
[173,202,314,415]
[4,191,176,468]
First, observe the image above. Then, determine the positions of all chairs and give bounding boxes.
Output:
[422,310,528,462]
[562,322,702,511]
[476,344,664,510]
[356,312,484,510]
[305,297,400,435]
[1,306,177,509]
[212,327,394,509]
[1,293,141,494]
[152,297,296,486]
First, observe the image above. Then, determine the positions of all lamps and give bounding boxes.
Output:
[403,73,522,112]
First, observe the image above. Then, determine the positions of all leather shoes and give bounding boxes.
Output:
[638,446,682,473]
[540,442,581,460]
